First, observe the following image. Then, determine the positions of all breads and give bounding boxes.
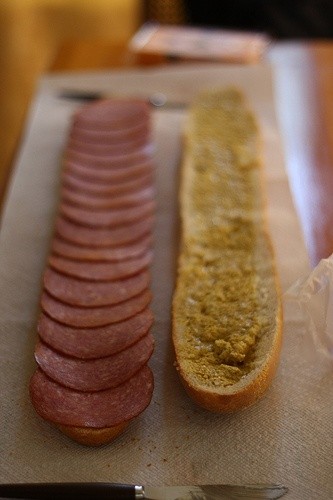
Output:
[169,86,280,410]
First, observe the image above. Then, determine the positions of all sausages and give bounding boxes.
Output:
[30,98,156,444]
